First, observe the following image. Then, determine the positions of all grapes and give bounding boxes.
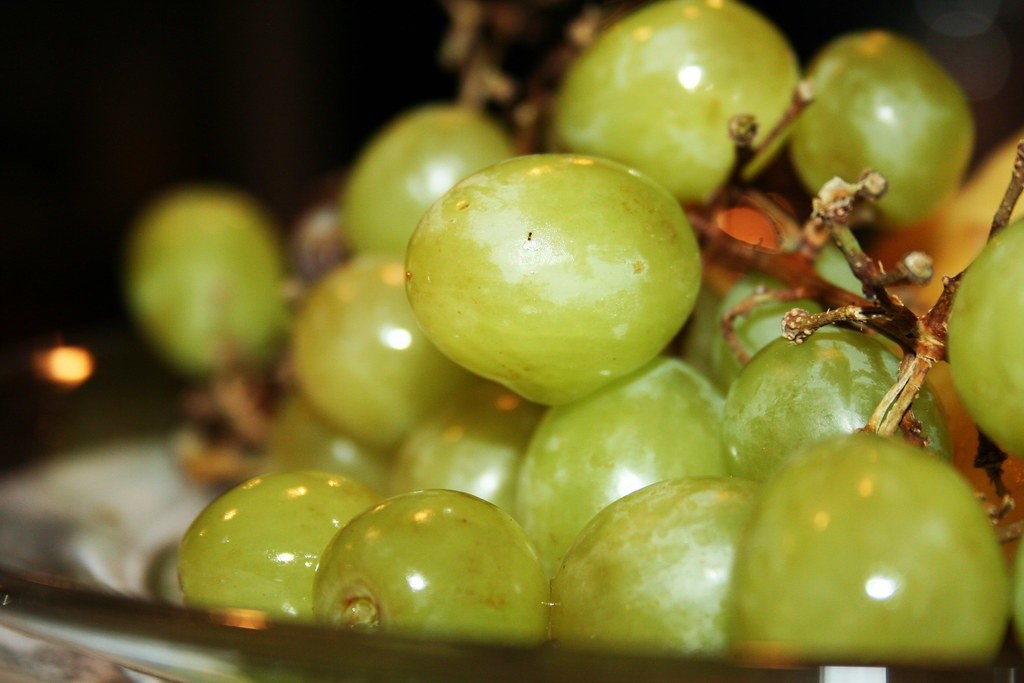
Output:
[125,1,1024,674]
